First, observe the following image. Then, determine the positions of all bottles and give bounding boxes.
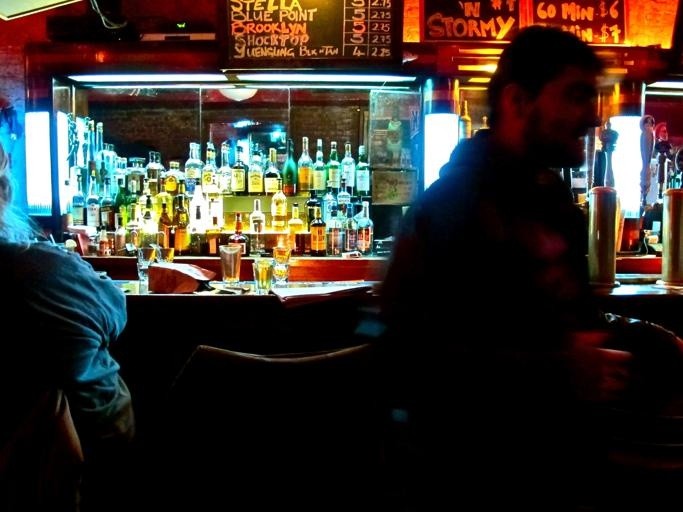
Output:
[478,115,489,131]
[456,99,470,143]
[67,136,375,257]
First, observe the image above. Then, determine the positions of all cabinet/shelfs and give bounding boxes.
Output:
[71,166,368,258]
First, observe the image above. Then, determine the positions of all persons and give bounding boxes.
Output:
[0,233,137,508]
[372,28,632,511]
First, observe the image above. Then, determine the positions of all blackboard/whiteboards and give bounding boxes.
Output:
[419,0,527,44]
[217,1,403,69]
[527,0,630,46]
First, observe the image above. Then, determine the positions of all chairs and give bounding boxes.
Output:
[162,342,377,511]
[0,380,111,512]
[598,316,683,511]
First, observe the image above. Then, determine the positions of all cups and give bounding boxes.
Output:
[252,259,273,292]
[216,244,241,283]
[157,247,173,262]
[585,186,616,282]
[659,188,683,283]
[134,247,154,280]
[270,245,289,286]
